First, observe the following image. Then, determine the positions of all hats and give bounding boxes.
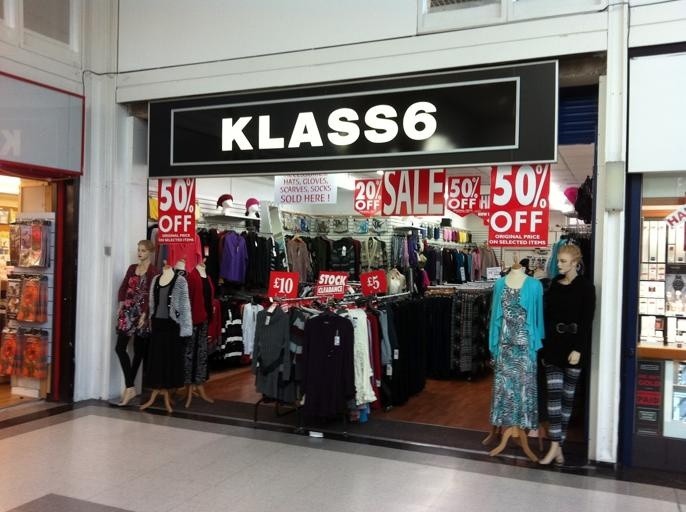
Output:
[217,194,233,206]
[245,197,259,208]
[564,187,578,204]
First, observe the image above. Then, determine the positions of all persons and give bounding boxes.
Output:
[486,262,546,463]
[138,264,193,412]
[244,198,260,218]
[214,192,235,215]
[112,237,159,407]
[172,257,187,278]
[536,242,597,468]
[183,262,217,409]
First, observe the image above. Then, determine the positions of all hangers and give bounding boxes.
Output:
[208,294,275,309]
[274,291,414,315]
[426,281,495,297]
[558,224,590,238]
[284,234,381,244]
[430,243,491,254]
[190,223,273,237]
